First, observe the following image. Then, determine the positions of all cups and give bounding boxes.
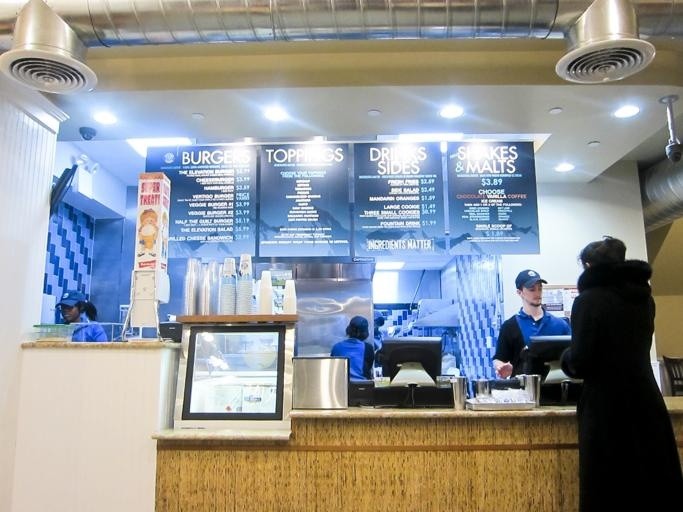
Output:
[517,374,540,409]
[451,377,467,409]
[259,270,273,313]
[475,379,489,399]
[283,279,297,315]
[183,254,253,316]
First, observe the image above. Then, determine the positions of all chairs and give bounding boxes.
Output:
[662,354,683,397]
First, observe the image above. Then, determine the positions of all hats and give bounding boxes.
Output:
[350,316,368,329]
[55,290,86,307]
[516,269,547,288]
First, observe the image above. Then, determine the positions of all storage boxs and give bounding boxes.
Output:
[32,323,74,343]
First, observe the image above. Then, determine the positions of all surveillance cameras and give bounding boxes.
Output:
[79,127,96,141]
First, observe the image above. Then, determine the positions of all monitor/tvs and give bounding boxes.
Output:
[529,336,573,380]
[381,337,442,387]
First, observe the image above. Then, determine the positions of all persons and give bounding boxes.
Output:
[331,316,375,381]
[560,237,683,511]
[55,290,107,342]
[374,310,385,376]
[492,270,572,379]
